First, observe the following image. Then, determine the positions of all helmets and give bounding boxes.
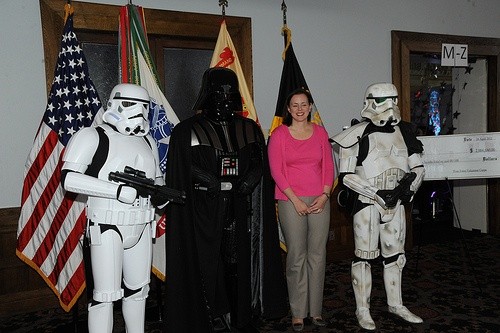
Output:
[360,82,401,127]
[102,83,150,137]
[192,67,243,112]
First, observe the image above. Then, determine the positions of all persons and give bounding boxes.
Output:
[60,83,168,333]
[164,66,268,333]
[328,83,425,330]
[266,90,334,330]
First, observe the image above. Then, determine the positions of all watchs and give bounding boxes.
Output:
[323,191,330,198]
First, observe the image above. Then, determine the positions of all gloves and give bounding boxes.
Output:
[228,179,252,197]
[393,173,417,205]
[117,184,137,204]
[377,190,399,207]
[150,195,169,215]
[202,177,220,200]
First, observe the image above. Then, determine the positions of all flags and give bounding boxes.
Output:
[265,25,340,251]
[123,4,182,281]
[15,5,105,313]
[200,19,262,129]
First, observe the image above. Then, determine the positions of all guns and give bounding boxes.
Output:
[107,167,187,205]
[385,172,416,209]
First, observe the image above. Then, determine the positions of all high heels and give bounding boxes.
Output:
[308,316,328,327]
[292,317,304,331]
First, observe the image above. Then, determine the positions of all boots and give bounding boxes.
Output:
[351,261,376,330]
[383,254,423,324]
[122,285,149,333]
[88,302,113,333]
